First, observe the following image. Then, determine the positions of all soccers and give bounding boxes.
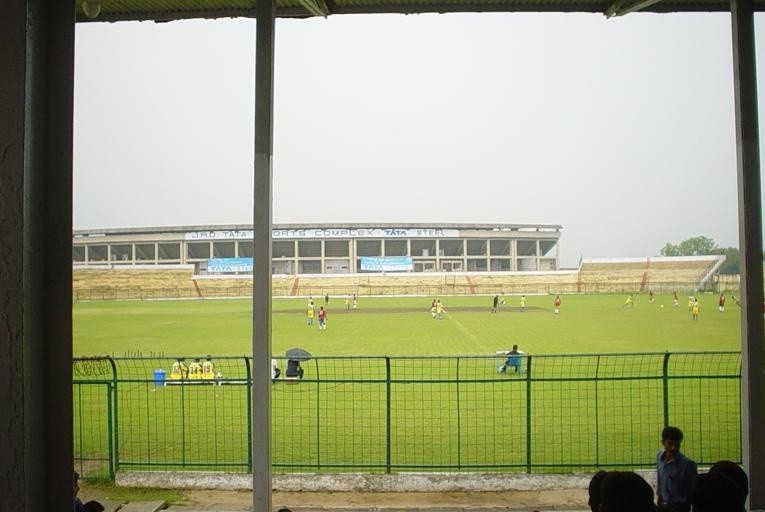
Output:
[661,304,664,307]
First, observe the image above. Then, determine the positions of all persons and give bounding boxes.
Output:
[492,295,499,313]
[187,356,204,379]
[82,499,104,512]
[73,471,84,511]
[499,291,506,306]
[586,426,749,512]
[519,295,527,311]
[429,297,438,318]
[285,359,305,379]
[437,300,448,318]
[554,295,562,314]
[306,293,357,331]
[622,288,742,321]
[495,344,525,374]
[169,357,188,380]
[203,355,223,381]
[271,356,280,385]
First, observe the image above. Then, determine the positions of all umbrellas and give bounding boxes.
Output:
[284,347,313,362]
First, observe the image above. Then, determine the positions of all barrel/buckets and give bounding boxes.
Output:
[153,370,166,385]
